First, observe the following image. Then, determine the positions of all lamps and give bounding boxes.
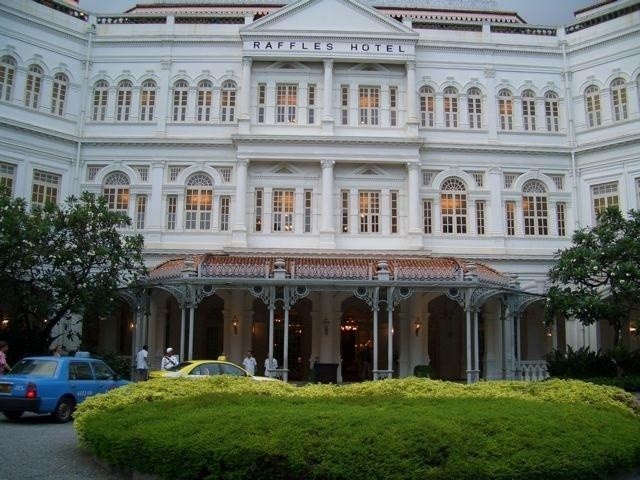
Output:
[129,319,137,335]
[323,316,330,336]
[415,317,422,336]
[232,314,239,335]
[341,314,359,331]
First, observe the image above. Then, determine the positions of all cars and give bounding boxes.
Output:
[148,355,282,381]
[0,352,136,424]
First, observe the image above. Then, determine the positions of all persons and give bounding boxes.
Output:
[160,347,180,370]
[53,345,65,356]
[242,351,258,377]
[134,345,150,381]
[263,352,279,377]
[360,349,372,381]
[217,352,228,361]
[0,341,12,375]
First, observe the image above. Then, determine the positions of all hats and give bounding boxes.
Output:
[166,346,173,354]
